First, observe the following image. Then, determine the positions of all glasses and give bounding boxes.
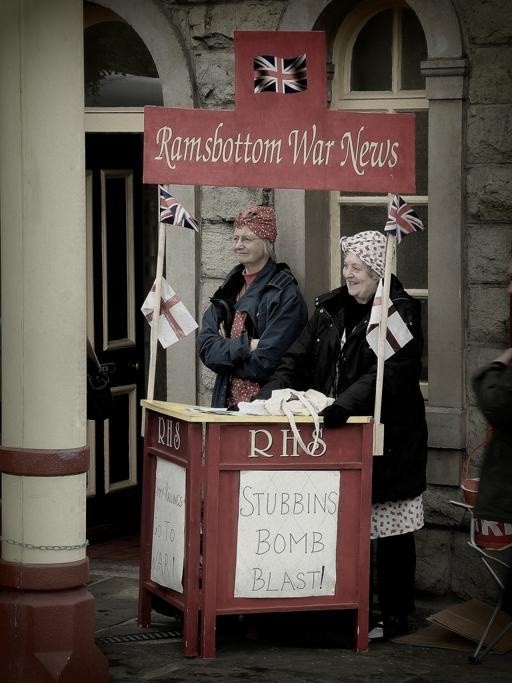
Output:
[231,235,272,243]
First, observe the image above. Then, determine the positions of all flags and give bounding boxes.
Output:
[383,194,427,244]
[160,185,200,234]
[363,277,414,363]
[140,273,200,350]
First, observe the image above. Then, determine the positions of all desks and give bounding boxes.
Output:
[137,399,372,660]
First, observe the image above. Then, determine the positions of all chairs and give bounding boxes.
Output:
[449,479,512,657]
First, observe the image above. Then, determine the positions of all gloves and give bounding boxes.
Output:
[318,404,350,428]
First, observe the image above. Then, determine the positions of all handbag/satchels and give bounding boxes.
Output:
[239,389,335,456]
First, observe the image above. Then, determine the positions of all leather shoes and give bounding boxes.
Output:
[368,621,408,638]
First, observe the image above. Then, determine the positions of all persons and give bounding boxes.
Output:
[469,331,512,615]
[248,229,431,644]
[196,204,310,411]
